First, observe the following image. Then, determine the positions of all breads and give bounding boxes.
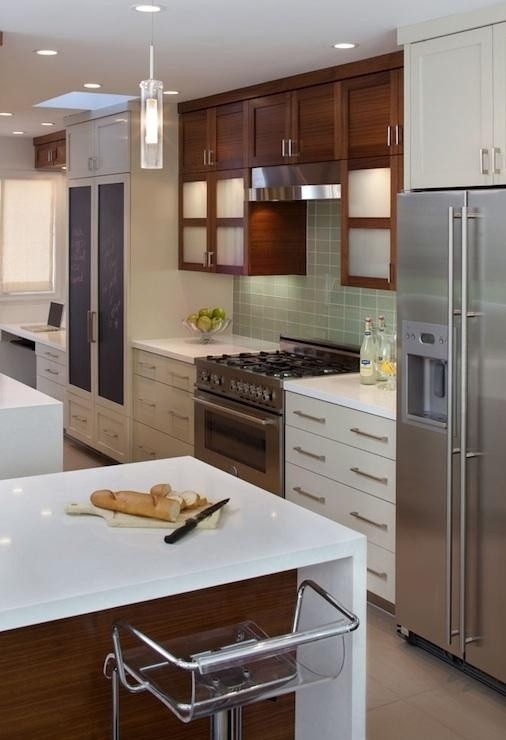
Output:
[91,484,209,522]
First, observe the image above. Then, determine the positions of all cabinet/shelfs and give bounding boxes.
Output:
[283,383,397,618]
[339,153,404,292]
[178,87,247,173]
[31,126,67,173]
[33,341,68,430]
[341,48,403,161]
[247,71,342,172]
[63,101,179,178]
[66,172,233,461]
[130,340,195,464]
[396,1,506,190]
[177,171,307,277]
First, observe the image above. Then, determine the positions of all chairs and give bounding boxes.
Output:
[103,580,361,740]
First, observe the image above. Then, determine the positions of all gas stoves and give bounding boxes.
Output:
[193,349,357,413]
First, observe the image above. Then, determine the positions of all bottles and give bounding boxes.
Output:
[358,315,391,385]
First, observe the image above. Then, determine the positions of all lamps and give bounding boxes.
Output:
[139,1,165,170]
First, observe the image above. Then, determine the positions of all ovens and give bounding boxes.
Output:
[192,390,284,498]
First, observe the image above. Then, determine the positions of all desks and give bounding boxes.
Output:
[0,372,65,481]
[1,455,369,739]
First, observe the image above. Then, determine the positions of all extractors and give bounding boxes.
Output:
[246,163,343,204]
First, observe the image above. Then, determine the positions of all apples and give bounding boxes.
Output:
[187,308,225,330]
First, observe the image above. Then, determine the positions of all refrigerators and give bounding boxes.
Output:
[392,189,506,691]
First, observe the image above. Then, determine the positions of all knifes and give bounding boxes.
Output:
[164,494,232,545]
